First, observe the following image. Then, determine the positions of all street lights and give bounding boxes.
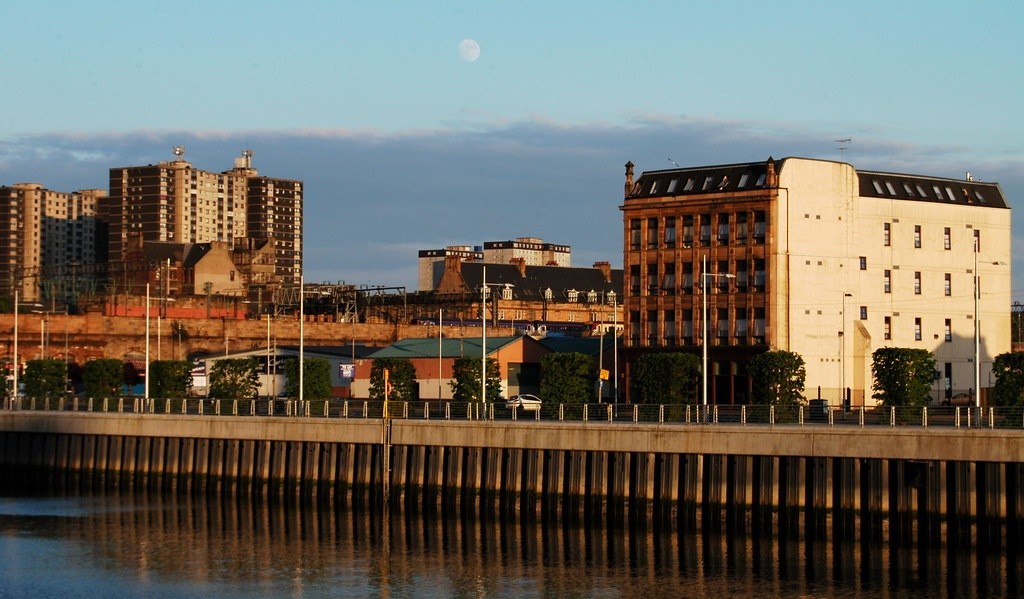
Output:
[11,288,43,402]
[299,274,331,416]
[480,263,515,421]
[702,254,737,424]
[145,283,177,412]
[972,238,1008,430]
